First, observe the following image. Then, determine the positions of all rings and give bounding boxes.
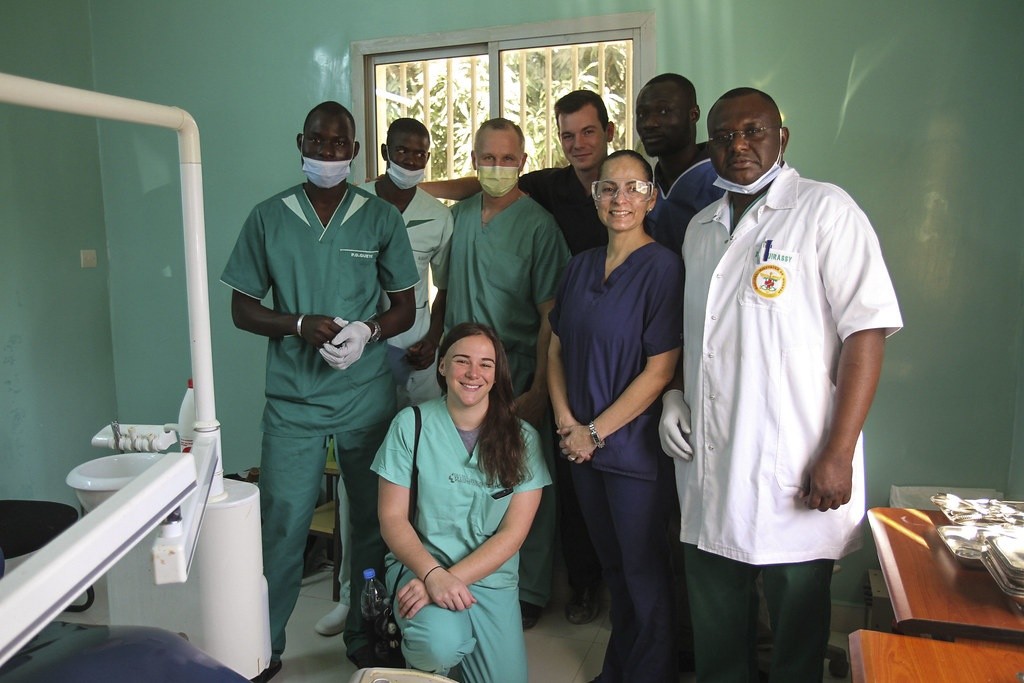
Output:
[568,455,576,461]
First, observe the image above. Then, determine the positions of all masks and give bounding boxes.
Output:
[475,165,518,198]
[384,146,425,191]
[300,136,356,190]
[712,162,783,196]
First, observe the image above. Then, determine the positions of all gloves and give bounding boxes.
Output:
[659,389,693,463]
[319,322,372,370]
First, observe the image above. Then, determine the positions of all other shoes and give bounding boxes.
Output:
[566,562,604,624]
[252,660,282,683]
[519,600,541,628]
[314,603,350,636]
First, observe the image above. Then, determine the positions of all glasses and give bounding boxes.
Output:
[590,179,654,203]
[707,124,779,144]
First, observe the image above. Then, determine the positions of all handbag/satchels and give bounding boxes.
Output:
[342,515,406,669]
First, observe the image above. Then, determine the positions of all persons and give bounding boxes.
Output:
[218,72,906,683]
[370,321,555,683]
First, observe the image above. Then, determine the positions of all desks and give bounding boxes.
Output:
[867,501,1024,641]
[847,629,1024,683]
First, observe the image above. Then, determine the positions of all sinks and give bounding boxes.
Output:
[66,452,166,512]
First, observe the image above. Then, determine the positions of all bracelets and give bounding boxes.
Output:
[423,565,443,584]
[296,313,306,338]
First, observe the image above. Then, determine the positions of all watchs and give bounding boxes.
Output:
[588,421,604,448]
[368,320,382,343]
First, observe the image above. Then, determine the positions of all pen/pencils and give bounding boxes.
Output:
[763,239,772,261]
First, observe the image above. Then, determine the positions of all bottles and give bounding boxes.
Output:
[178,379,194,454]
[361,568,387,619]
